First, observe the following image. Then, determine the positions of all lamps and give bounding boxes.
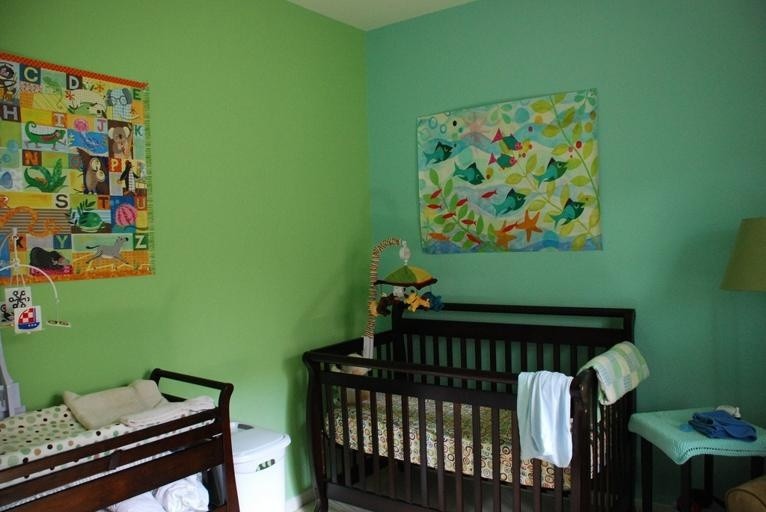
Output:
[720,217,766,291]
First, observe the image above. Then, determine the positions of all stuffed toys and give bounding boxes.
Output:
[369,240,443,315]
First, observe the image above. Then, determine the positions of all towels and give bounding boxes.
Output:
[575,341,650,423]
[688,411,757,443]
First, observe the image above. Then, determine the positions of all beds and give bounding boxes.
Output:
[303,294,636,512]
[0,368,240,512]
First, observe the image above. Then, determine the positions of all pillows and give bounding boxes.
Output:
[63,380,214,431]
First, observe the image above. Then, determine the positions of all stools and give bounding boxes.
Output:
[627,407,766,512]
